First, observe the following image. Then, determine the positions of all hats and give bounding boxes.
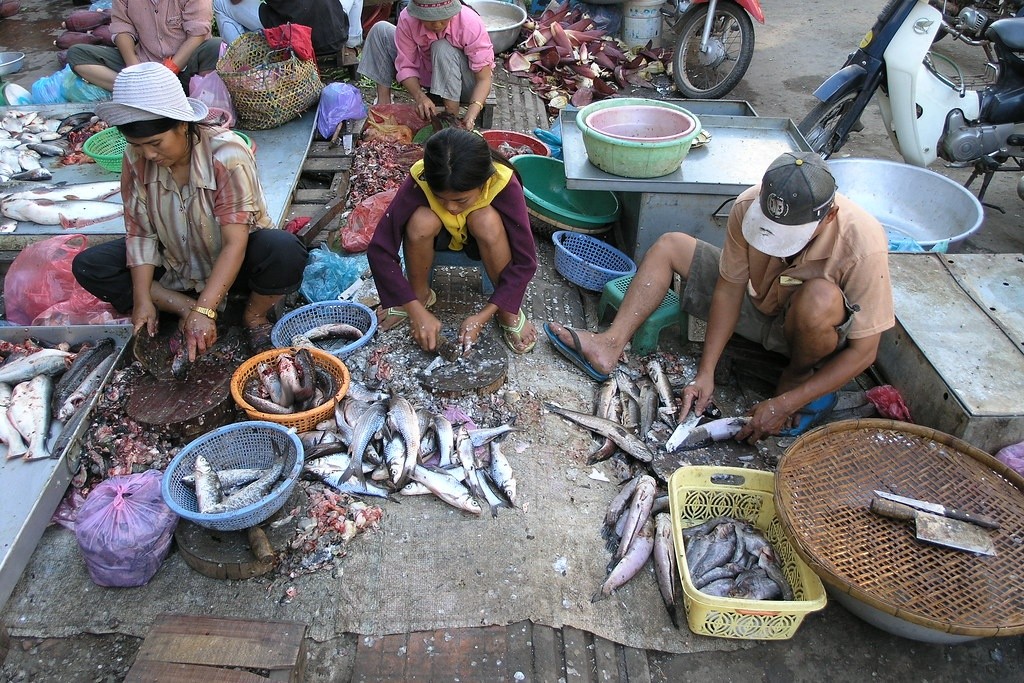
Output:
[740,150,839,258]
[93,62,209,130]
[405,0,464,21]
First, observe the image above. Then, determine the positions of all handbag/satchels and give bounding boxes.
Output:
[190,68,236,130]
[317,81,367,139]
[4,234,122,327]
[73,469,179,587]
[340,186,399,252]
[216,17,326,130]
[19,63,111,105]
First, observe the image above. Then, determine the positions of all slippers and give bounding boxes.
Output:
[776,391,840,436]
[495,308,539,355]
[374,288,437,330]
[542,321,608,383]
[241,313,276,354]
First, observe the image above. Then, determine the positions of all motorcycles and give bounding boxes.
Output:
[660,0,763,98]
[796,0,1023,215]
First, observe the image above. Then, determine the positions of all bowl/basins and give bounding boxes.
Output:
[574,97,701,178]
[824,159,985,254]
[586,107,695,143]
[467,1,527,54]
[0,51,25,76]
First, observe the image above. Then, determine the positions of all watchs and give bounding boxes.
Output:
[189,303,219,320]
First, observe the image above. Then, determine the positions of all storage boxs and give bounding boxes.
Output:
[559,96,818,280]
[889,252,1024,459]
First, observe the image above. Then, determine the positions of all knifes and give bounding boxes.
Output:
[132,302,169,380]
[874,489,1001,529]
[871,497,996,556]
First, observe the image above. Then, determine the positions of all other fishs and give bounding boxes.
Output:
[289,322,364,351]
[0,107,129,234]
[589,472,683,633]
[301,391,523,522]
[681,510,795,606]
[182,435,290,514]
[0,335,120,462]
[543,357,756,489]
[170,333,221,383]
[243,347,338,416]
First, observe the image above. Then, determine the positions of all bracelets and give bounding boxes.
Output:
[468,100,485,110]
[160,56,180,75]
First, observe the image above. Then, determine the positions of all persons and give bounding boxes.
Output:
[540,152,896,446]
[366,126,540,355]
[355,0,496,139]
[66,0,228,104]
[70,59,308,364]
[213,0,365,67]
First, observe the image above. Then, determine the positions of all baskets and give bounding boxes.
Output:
[552,230,637,293]
[203,106,232,130]
[229,347,350,433]
[84,127,130,173]
[160,420,305,533]
[666,462,827,640]
[271,300,377,363]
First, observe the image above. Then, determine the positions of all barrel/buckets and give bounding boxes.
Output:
[621,0,667,48]
